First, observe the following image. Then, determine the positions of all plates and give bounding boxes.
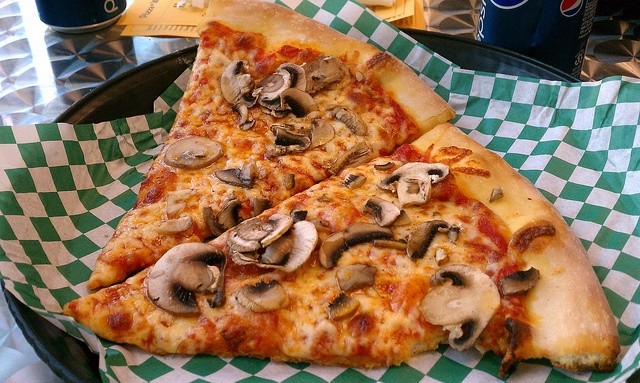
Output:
[3,26,639,383]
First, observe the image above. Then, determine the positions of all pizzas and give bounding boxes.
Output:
[61,123,609,370]
[60,0,453,286]
